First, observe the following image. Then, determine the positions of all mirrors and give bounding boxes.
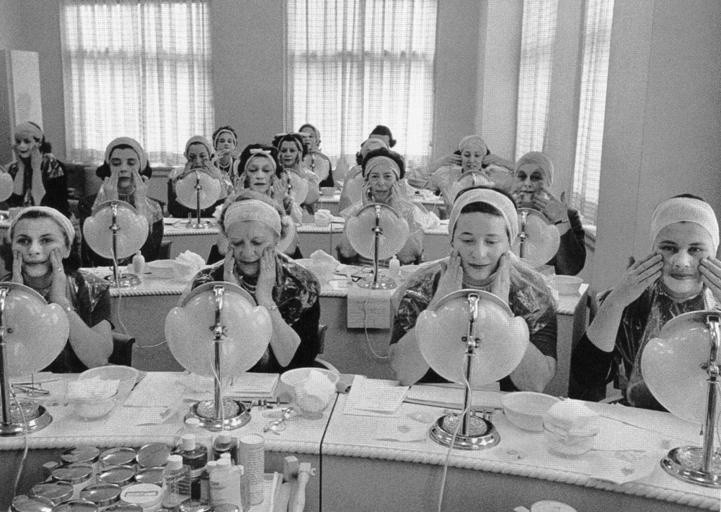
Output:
[344,203,408,290]
[511,209,560,269]
[284,169,307,205]
[164,281,273,432]
[302,151,331,182]
[82,200,149,288]
[455,169,493,193]
[415,289,530,450]
[0,165,14,204]
[0,281,71,436]
[641,310,721,489]
[175,170,219,230]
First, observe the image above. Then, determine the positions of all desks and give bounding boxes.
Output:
[320,371,721,512]
[0,262,590,397]
[311,189,447,218]
[0,372,338,512]
[0,217,451,266]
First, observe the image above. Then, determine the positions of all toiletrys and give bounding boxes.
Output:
[143,430,277,511]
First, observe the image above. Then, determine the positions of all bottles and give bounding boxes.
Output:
[132,251,145,281]
[161,431,266,512]
[389,255,400,277]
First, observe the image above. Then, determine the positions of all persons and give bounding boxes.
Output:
[389,183,561,396]
[1,207,114,375]
[1,123,587,299]
[175,200,324,369]
[567,194,721,403]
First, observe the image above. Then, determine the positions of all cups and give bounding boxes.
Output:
[514,500,578,512]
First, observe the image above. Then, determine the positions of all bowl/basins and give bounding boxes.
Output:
[321,187,337,199]
[294,389,331,419]
[400,265,420,275]
[501,391,562,432]
[66,389,119,419]
[554,276,583,295]
[280,367,340,401]
[146,260,179,278]
[78,365,140,404]
[314,212,330,227]
[541,421,600,457]
